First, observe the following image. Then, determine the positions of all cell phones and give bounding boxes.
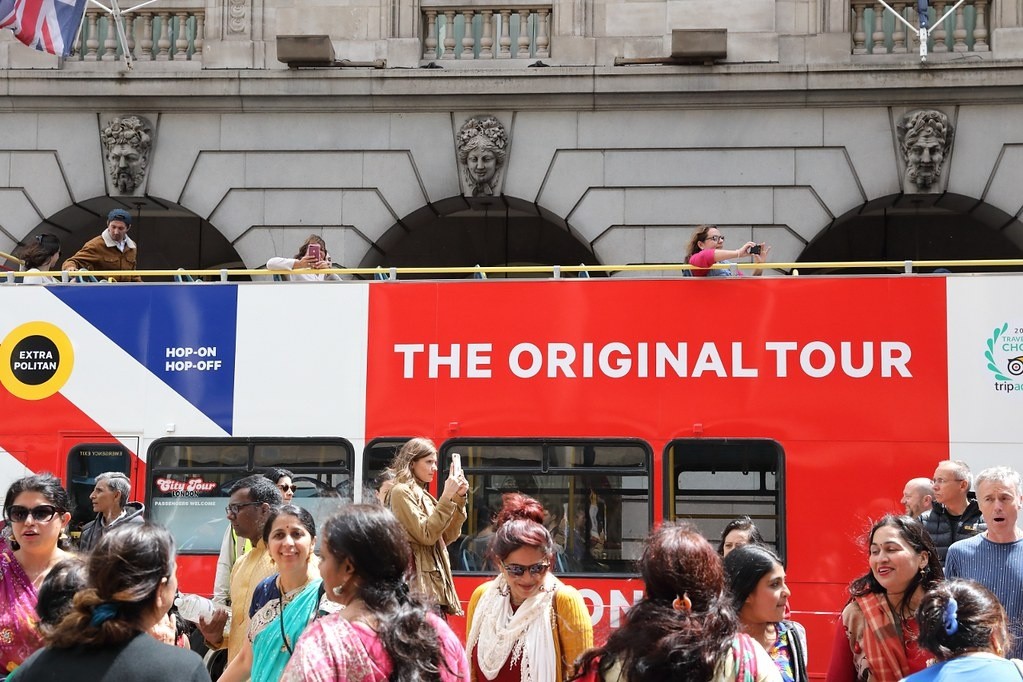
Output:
[452,454,461,478]
[309,244,320,264]
[749,245,761,254]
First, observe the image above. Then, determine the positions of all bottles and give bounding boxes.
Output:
[174,595,232,634]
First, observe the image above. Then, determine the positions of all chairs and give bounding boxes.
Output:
[158,474,390,498]
[460,533,573,573]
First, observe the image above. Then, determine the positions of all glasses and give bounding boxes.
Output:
[934,478,964,484]
[225,501,270,514]
[6,505,70,523]
[278,485,296,493]
[500,560,551,578]
[58,251,62,256]
[705,235,725,242]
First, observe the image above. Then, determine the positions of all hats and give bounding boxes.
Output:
[108,209,131,225]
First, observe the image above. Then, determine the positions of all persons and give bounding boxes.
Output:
[456,114,509,197]
[468,491,589,572]
[0,471,213,682]
[15,209,144,285]
[266,233,344,282]
[465,492,593,682]
[561,514,809,682]
[372,436,470,624]
[898,109,955,193]
[192,467,471,682]
[682,223,772,278]
[826,460,1023,682]
[102,115,153,195]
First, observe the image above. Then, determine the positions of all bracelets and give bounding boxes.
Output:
[736,249,740,258]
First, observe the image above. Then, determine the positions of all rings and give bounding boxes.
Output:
[308,263,311,265]
